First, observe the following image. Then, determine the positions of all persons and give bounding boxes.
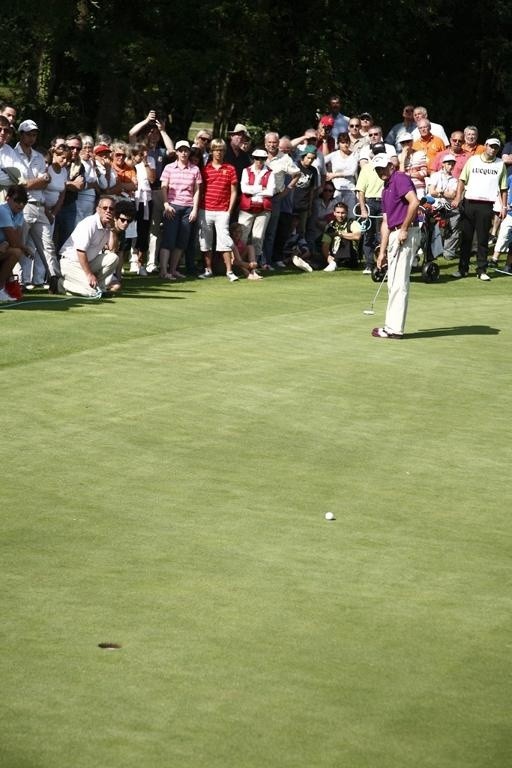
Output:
[369,154,422,339]
[0,94,512,304]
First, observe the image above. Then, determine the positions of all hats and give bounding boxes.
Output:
[398,132,413,144]
[93,144,111,154]
[299,145,317,156]
[17,119,38,133]
[370,151,394,170]
[229,123,253,142]
[360,112,372,118]
[443,154,456,163]
[175,140,191,150]
[485,138,501,146]
[252,149,269,158]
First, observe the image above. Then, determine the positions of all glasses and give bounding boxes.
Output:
[0,127,9,133]
[369,132,378,137]
[349,124,359,129]
[119,217,133,224]
[97,205,113,212]
[10,196,28,204]
[198,136,211,144]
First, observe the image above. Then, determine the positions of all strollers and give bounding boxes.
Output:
[366,198,441,281]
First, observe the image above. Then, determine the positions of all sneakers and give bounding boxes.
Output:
[22,283,52,293]
[130,261,190,281]
[324,260,338,272]
[0,291,17,305]
[489,260,512,275]
[226,271,239,282]
[372,327,404,338]
[363,266,375,274]
[452,271,468,278]
[476,272,491,281]
[262,260,287,271]
[198,267,213,279]
[247,270,264,281]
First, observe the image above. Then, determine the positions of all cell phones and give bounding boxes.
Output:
[151,111,157,122]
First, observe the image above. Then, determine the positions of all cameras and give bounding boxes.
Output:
[39,175,49,181]
[325,126,330,134]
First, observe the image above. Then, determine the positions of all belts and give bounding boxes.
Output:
[59,255,66,260]
[28,201,45,207]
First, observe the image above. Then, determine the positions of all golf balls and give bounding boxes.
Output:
[324,511,335,520]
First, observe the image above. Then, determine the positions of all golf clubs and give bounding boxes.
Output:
[363,244,403,316]
